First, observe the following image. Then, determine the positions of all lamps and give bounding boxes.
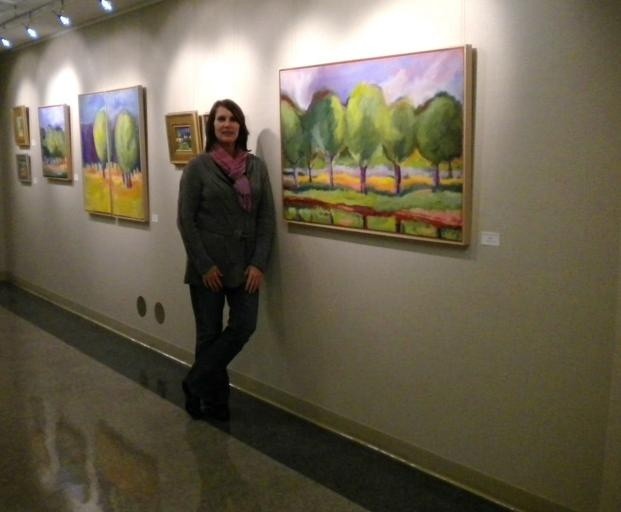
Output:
[1,1,114,49]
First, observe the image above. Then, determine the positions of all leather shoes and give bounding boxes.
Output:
[182,380,202,419]
[212,404,229,421]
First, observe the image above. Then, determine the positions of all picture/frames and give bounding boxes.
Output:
[165,111,203,166]
[278,44,472,251]
[39,104,73,182]
[11,105,31,147]
[76,83,151,224]
[15,153,33,184]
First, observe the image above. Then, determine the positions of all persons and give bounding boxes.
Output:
[174,97,278,425]
